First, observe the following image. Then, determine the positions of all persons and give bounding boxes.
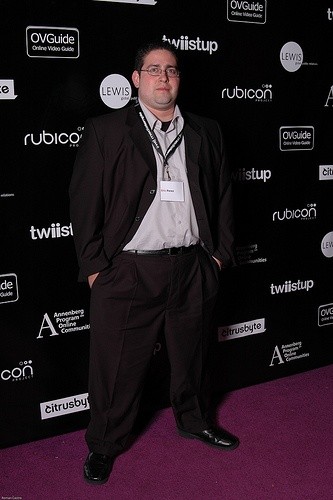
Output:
[69,38,241,484]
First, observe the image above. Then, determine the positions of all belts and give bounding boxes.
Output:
[123,244,201,256]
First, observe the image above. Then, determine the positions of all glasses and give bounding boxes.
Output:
[136,67,179,77]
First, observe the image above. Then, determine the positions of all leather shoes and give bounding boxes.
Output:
[178,424,239,449]
[82,450,116,483]
